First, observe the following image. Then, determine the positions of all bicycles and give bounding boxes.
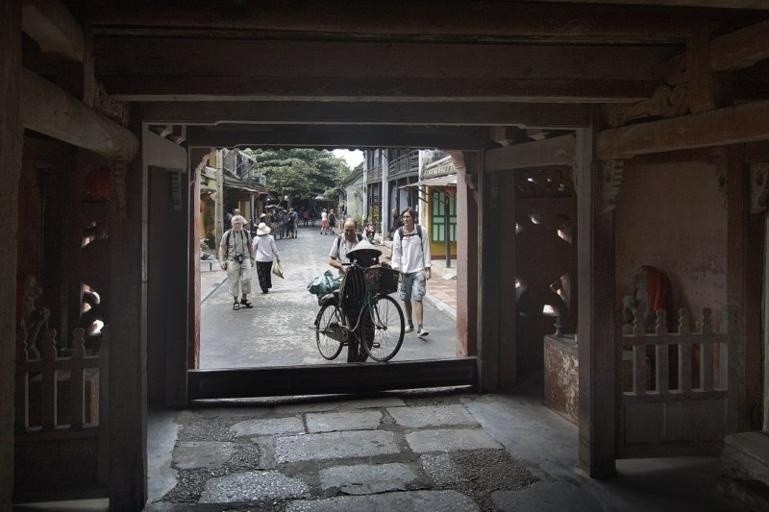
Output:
[311,260,406,362]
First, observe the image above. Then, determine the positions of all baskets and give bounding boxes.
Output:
[366,267,399,292]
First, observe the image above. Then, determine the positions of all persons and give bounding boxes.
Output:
[329,218,369,279]
[391,207,431,337]
[386,208,404,261]
[277,205,338,239]
[365,223,376,245]
[339,238,383,362]
[233,208,240,215]
[252,222,280,293]
[219,215,254,310]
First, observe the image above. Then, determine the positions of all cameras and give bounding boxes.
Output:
[233,253,243,264]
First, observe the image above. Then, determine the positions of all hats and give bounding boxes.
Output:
[346,239,382,258]
[232,216,241,223]
[257,223,271,235]
[273,263,284,279]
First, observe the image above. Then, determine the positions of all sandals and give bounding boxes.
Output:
[240,298,253,308]
[233,302,239,310]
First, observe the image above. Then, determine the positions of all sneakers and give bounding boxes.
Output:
[405,325,415,333]
[417,330,429,337]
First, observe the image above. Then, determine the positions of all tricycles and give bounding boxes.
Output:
[264,219,284,241]
